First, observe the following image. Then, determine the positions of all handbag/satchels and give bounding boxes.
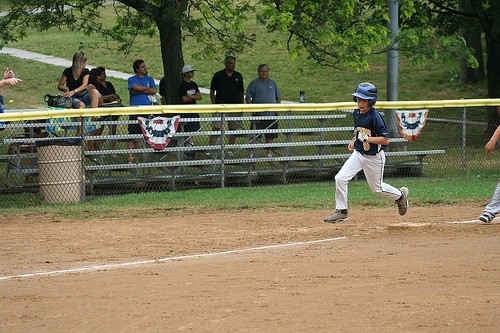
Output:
[44,94,73,108]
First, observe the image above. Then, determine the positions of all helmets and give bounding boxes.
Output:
[352,82,378,106]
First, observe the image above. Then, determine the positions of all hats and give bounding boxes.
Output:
[182,65,197,73]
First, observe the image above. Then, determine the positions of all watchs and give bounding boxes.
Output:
[74,90,77,93]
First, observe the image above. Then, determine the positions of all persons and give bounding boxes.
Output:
[210,56,244,158]
[0,68,23,131]
[127,60,157,172]
[58,51,103,137]
[245,64,281,158]
[88,66,122,159]
[159,76,167,117]
[323,82,409,223]
[176,65,202,154]
[479,125,500,223]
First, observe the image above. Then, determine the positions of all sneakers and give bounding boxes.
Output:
[479,215,492,223]
[324,210,349,223]
[395,186,409,215]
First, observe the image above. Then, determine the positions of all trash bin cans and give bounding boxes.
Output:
[35,138,85,204]
